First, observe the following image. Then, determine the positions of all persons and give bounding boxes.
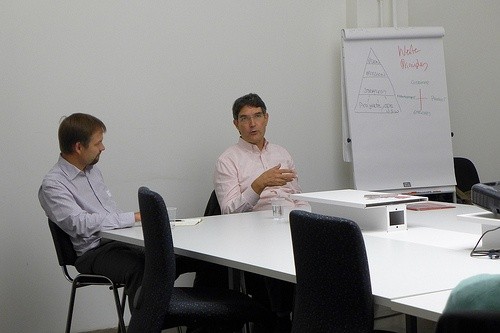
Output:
[213,92,310,304]
[39,113,226,290]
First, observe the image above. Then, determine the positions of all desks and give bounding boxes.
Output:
[98,201,500,323]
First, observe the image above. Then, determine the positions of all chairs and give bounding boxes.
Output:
[127,187,255,333]
[454,157,497,204]
[48,217,130,333]
[290,210,399,333]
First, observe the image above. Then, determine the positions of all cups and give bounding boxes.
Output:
[166,207,176,231]
[270,198,285,220]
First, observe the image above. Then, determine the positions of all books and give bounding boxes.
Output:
[169,218,202,226]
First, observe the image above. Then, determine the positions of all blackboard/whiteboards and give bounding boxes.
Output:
[341,27,457,195]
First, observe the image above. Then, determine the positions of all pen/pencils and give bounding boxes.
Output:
[170,220,181,221]
